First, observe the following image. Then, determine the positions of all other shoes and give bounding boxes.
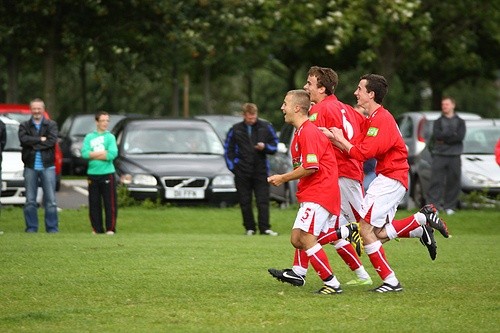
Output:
[246,230,256,237]
[264,230,278,236]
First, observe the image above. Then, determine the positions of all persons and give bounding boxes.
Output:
[267,89,364,295]
[268,66,439,287]
[494,138,500,167]
[0,119,7,234]
[18,98,58,234]
[427,96,465,214]
[223,102,278,236]
[318,74,449,293]
[81,111,118,234]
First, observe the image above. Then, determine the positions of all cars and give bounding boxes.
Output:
[394,109,500,209]
[56,111,130,176]
[193,113,288,157]
[0,104,63,193]
[111,115,239,208]
[0,116,44,205]
[268,119,298,209]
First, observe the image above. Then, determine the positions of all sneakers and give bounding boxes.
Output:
[371,283,403,294]
[419,223,437,260]
[346,222,363,256]
[317,285,343,295]
[420,204,449,239]
[268,269,307,287]
[346,276,373,286]
